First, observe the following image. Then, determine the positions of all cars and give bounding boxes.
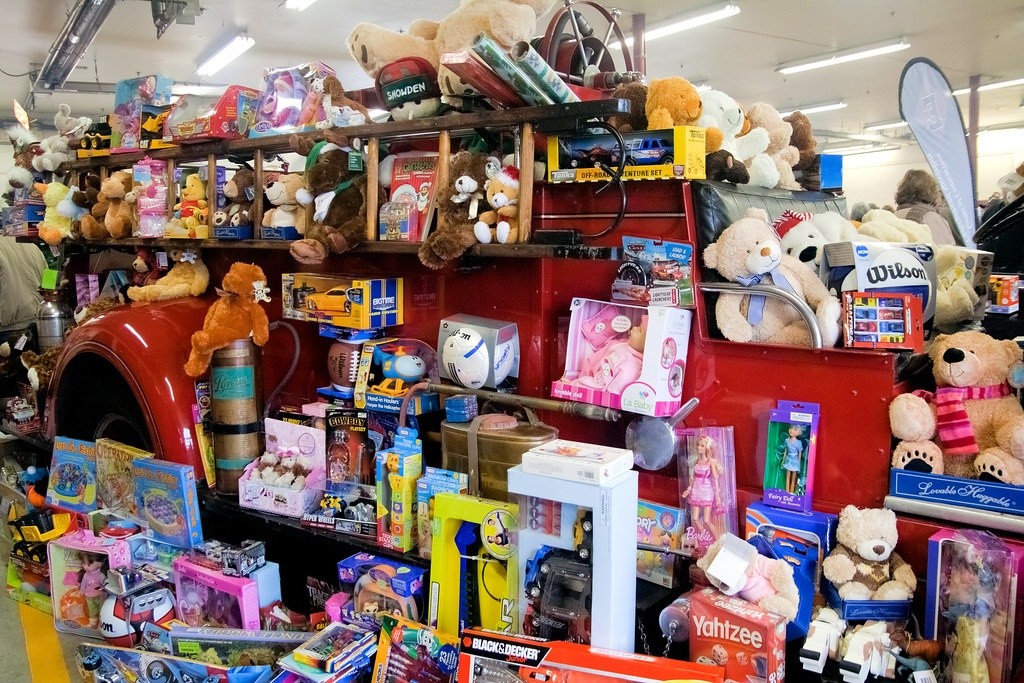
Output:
[523,606,541,635]
[853,296,906,345]
[569,147,608,169]
[573,509,592,564]
[536,556,594,643]
[304,284,352,313]
[523,545,554,597]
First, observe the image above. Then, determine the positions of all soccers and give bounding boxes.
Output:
[442,328,515,389]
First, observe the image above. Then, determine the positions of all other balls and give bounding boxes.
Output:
[826,248,930,315]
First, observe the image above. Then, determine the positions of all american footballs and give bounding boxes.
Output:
[327,341,362,392]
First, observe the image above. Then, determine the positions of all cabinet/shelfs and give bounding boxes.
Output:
[58,96,618,257]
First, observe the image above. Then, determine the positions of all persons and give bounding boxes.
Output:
[0,233,52,395]
[892,168,961,247]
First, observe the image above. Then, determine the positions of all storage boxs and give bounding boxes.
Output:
[378,192,419,241]
[45,273,915,683]
[3,188,48,236]
[547,124,706,183]
[957,246,1022,324]
[925,528,1024,671]
[881,466,1024,517]
[164,83,264,144]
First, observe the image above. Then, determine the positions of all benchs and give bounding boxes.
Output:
[694,178,939,450]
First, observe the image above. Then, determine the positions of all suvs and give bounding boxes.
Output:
[79,122,113,149]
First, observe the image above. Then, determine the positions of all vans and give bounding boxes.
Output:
[608,137,674,167]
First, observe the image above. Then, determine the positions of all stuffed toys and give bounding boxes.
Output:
[4,4,1022,683]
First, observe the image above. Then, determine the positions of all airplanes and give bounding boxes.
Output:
[372,345,428,384]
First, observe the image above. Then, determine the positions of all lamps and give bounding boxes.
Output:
[197,32,256,77]
[825,142,902,156]
[775,36,911,75]
[778,97,848,119]
[607,1,741,50]
[862,118,909,131]
[28,0,118,95]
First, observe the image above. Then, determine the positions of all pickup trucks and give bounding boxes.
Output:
[7,497,77,568]
